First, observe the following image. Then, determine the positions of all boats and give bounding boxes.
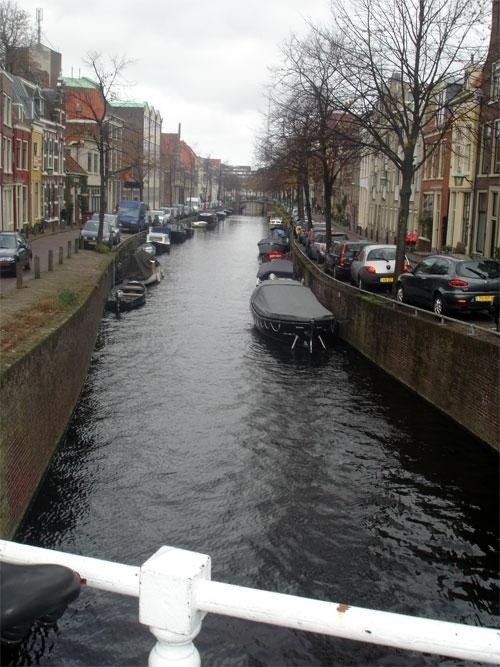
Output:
[135,242,156,257]
[108,278,146,313]
[117,251,164,287]
[167,204,234,245]
[250,276,338,355]
[256,210,299,283]
[145,224,172,254]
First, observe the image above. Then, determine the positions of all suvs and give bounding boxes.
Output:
[324,240,378,280]
[306,232,348,263]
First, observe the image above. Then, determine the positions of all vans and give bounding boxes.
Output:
[87,212,124,245]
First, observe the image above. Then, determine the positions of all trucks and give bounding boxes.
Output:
[116,200,145,233]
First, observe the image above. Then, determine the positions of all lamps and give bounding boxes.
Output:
[452,175,473,189]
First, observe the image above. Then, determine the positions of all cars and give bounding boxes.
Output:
[79,220,113,250]
[351,244,411,293]
[146,197,224,225]
[270,196,335,245]
[398,253,500,319]
[0,231,32,277]
